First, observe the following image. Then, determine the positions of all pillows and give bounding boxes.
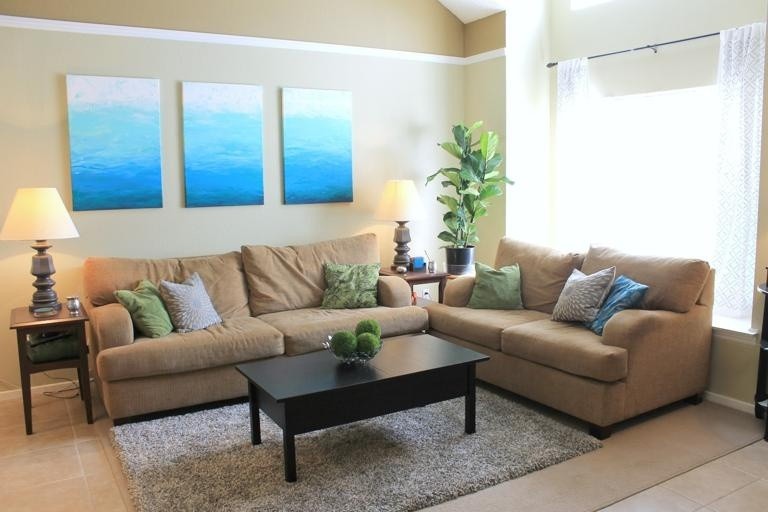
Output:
[582,275,649,335]
[550,265,617,323]
[154,270,222,334]
[113,277,174,339]
[466,262,524,311]
[321,259,383,310]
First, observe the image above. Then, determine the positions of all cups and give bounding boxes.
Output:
[66,297,80,316]
[428,261,436,273]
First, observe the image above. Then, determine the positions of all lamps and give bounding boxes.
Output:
[372,179,427,270]
[0,187,79,314]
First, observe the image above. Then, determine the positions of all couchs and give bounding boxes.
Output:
[79,232,430,426]
[423,235,715,440]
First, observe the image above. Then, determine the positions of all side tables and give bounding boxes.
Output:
[378,264,448,307]
[8,301,95,435]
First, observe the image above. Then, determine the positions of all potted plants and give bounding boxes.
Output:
[426,118,513,276]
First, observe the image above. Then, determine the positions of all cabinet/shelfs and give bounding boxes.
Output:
[753,283,768,443]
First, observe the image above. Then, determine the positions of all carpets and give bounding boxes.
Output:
[106,377,604,512]
